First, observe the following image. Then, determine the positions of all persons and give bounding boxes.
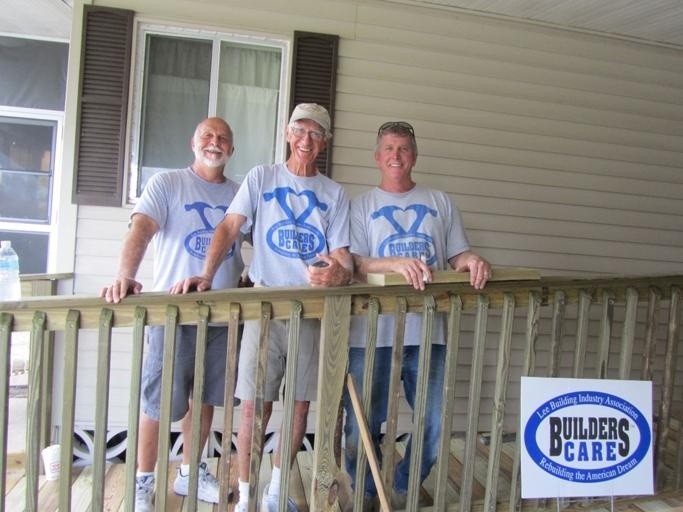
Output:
[165,101,356,510]
[341,122,491,511]
[97,116,257,511]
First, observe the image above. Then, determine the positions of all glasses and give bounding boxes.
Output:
[290,124,326,143]
[377,121,414,136]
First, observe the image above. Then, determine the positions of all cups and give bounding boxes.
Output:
[42,446,60,482]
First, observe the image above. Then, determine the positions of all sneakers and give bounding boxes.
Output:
[391,481,423,512]
[172,465,232,505]
[355,490,381,512]
[230,498,258,512]
[262,484,299,512]
[129,474,155,512]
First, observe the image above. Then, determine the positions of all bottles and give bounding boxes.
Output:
[0,240,21,301]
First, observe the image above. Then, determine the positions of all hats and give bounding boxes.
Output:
[288,102,331,140]
